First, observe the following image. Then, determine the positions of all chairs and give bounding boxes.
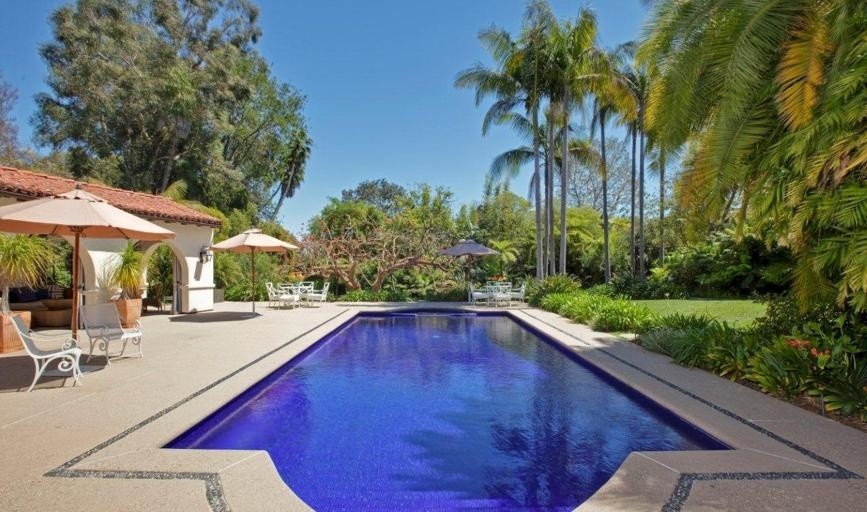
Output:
[6,311,88,393]
[140,280,165,313]
[462,279,529,311]
[76,301,144,365]
[263,279,329,311]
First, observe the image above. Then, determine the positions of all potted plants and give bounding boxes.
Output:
[108,236,146,328]
[0,229,50,356]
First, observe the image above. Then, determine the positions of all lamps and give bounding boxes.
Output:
[196,243,213,265]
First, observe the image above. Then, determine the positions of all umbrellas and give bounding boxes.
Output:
[439,240,498,285]
[206,227,299,317]
[0,181,177,381]
